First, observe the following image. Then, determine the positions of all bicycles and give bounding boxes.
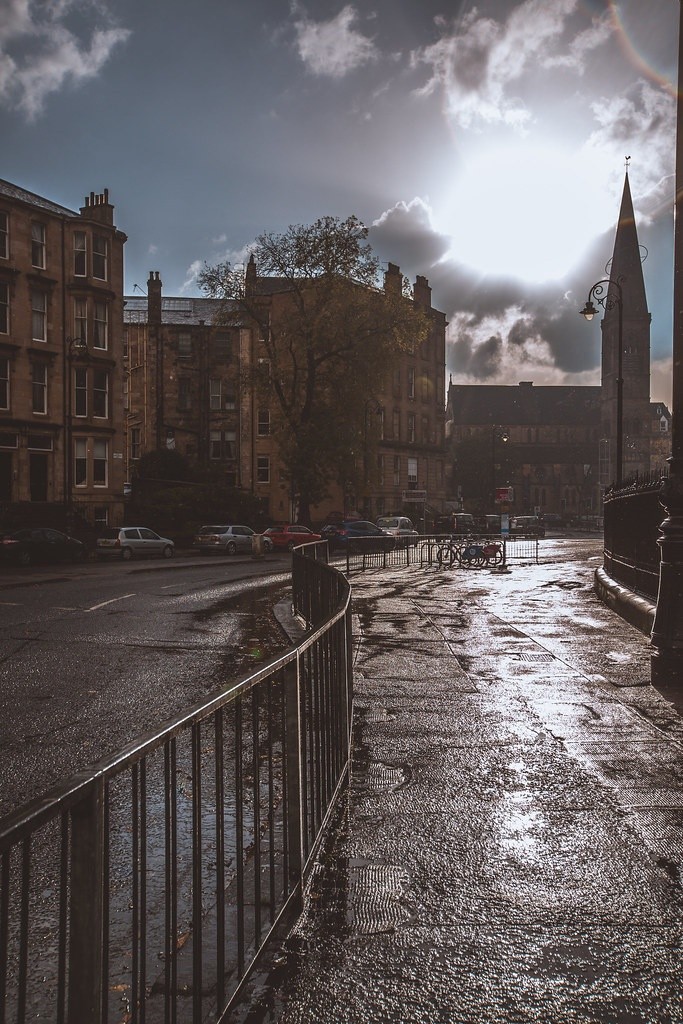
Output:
[437,533,502,567]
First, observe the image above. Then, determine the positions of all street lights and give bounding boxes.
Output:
[67,336,94,507]
[363,399,384,522]
[491,426,510,515]
[579,274,626,489]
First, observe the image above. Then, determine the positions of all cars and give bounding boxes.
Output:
[0,528,92,564]
[432,513,475,542]
[263,525,322,552]
[192,525,273,555]
[376,517,419,547]
[96,527,174,560]
[515,516,544,534]
[319,521,397,554]
